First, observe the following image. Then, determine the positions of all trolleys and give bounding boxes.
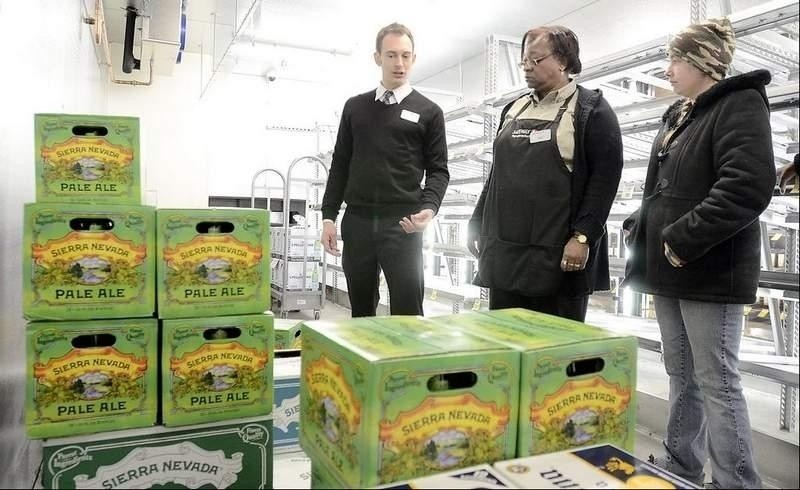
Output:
[250,156,329,321]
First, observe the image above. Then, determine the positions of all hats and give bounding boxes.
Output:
[669,16,736,82]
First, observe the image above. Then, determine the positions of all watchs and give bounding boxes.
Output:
[570,231,589,244]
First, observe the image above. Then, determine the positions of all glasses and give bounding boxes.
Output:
[518,53,552,68]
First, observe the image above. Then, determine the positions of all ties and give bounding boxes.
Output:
[383,91,394,104]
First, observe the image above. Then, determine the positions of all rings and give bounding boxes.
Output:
[568,262,574,268]
[559,259,567,269]
[576,263,580,268]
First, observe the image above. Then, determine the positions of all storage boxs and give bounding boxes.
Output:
[497,442,706,486]
[377,463,514,490]
[297,316,518,485]
[22,112,276,489]
[433,306,641,456]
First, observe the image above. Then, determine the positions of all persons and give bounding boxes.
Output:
[776,153,799,194]
[623,16,775,489]
[320,22,450,318]
[465,26,624,322]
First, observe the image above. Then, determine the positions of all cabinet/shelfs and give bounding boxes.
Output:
[486,3,799,426]
[309,96,481,313]
